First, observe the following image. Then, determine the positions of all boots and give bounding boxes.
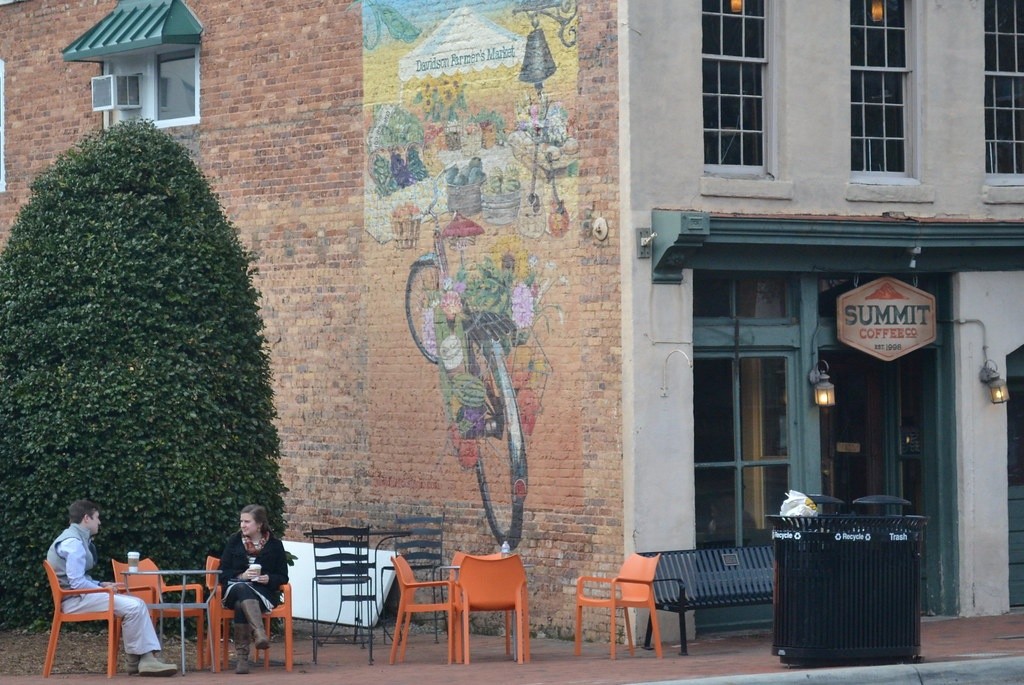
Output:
[234,623,250,673]
[241,599,270,650]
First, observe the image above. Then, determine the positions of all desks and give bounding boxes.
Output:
[439,563,534,661]
[302,528,393,642]
[118,570,224,675]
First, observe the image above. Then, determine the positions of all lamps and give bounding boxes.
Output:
[906,245,923,269]
[809,359,835,408]
[730,0,742,13]
[872,0,883,21]
[979,359,1012,406]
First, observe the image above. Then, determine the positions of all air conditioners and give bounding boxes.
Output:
[92,73,143,111]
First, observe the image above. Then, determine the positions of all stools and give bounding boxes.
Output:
[375,511,446,645]
[310,526,377,668]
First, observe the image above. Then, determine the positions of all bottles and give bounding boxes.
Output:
[501,540,511,559]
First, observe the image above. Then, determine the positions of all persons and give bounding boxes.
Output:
[46,498,178,676]
[218,503,289,674]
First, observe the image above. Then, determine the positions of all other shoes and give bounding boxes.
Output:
[126,662,139,672]
[139,658,177,676]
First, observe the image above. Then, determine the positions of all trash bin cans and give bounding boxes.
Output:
[765,494,929,668]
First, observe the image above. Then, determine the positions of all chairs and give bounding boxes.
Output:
[573,552,664,660]
[390,555,463,665]
[41,559,120,679]
[449,551,510,658]
[454,553,530,665]
[205,555,295,674]
[112,557,205,668]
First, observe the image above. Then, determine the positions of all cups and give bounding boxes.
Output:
[250,564,262,582]
[127,551,140,572]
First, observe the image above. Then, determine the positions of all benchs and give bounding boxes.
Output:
[637,543,774,655]
